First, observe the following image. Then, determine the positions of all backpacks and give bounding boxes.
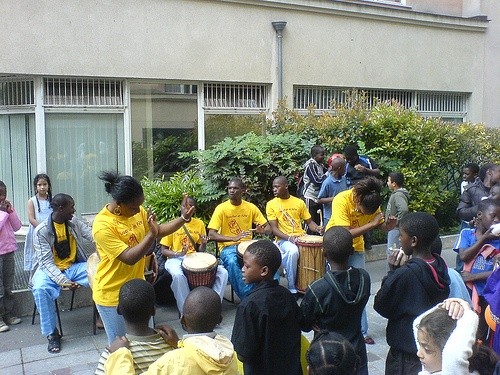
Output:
[295,163,318,197]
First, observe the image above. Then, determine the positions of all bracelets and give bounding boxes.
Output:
[181,214,191,222]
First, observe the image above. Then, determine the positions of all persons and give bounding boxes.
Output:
[453,163,500,340]
[0,181,22,333]
[24,174,93,289]
[92,169,195,347]
[385,172,410,272]
[94,278,176,375]
[31,176,325,353]
[413,297,499,375]
[373,212,451,375]
[305,329,361,375]
[482,253,500,355]
[231,239,305,375]
[303,145,380,236]
[300,226,371,375]
[431,237,473,310]
[104,286,244,375]
[324,175,398,344]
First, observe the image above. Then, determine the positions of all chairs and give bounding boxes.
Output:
[32,287,97,337]
[213,240,234,304]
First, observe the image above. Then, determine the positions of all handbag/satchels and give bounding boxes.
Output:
[56,239,71,259]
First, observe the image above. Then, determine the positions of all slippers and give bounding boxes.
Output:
[47,327,62,353]
[97,326,105,330]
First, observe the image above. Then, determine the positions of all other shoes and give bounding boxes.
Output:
[364,245,372,250]
[364,336,375,344]
[293,293,299,301]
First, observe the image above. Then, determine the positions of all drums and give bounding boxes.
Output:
[181,251,218,290]
[298,233,327,294]
[235,239,261,265]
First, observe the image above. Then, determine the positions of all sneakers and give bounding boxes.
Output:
[0,320,9,332]
[7,317,22,324]
